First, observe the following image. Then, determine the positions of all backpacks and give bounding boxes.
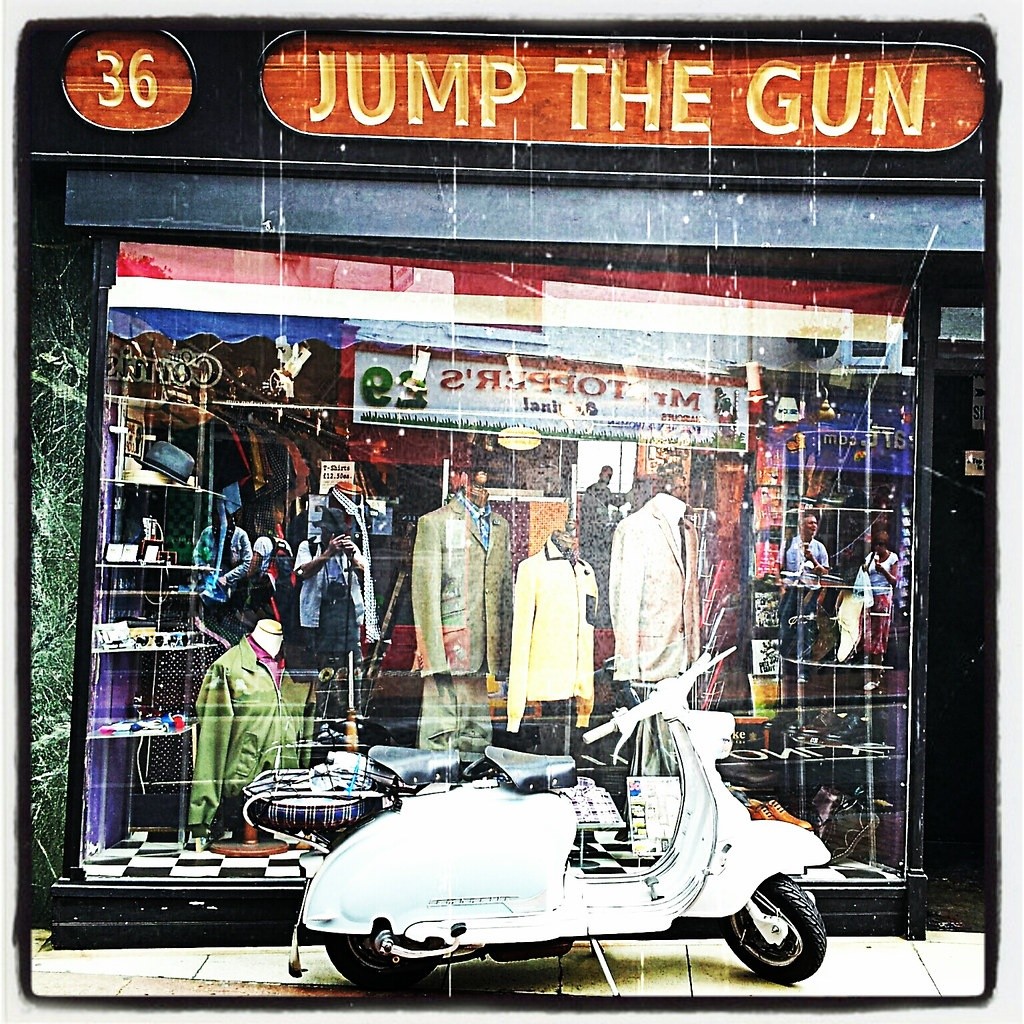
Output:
[245,534,300,622]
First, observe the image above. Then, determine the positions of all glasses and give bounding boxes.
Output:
[870,541,891,548]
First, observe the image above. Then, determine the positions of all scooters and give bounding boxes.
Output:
[301,642,833,994]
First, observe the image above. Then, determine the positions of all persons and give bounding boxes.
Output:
[610,492,701,766]
[578,467,614,532]
[293,507,370,669]
[411,485,512,752]
[858,524,901,689]
[776,514,829,687]
[507,529,597,754]
[187,620,306,841]
[194,510,294,617]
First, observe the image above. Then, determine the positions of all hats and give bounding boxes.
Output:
[309,507,355,534]
[132,440,196,488]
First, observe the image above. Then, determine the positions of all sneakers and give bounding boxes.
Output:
[795,673,809,683]
[863,681,880,691]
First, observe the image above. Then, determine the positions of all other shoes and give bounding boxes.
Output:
[811,602,839,661]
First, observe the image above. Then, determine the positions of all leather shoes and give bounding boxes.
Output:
[812,786,880,864]
[834,589,867,663]
[802,709,873,745]
[745,799,811,830]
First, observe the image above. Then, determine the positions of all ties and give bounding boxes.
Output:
[331,481,382,646]
[456,485,492,551]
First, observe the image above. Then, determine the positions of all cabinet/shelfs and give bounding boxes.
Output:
[89,395,229,858]
[764,367,898,865]
